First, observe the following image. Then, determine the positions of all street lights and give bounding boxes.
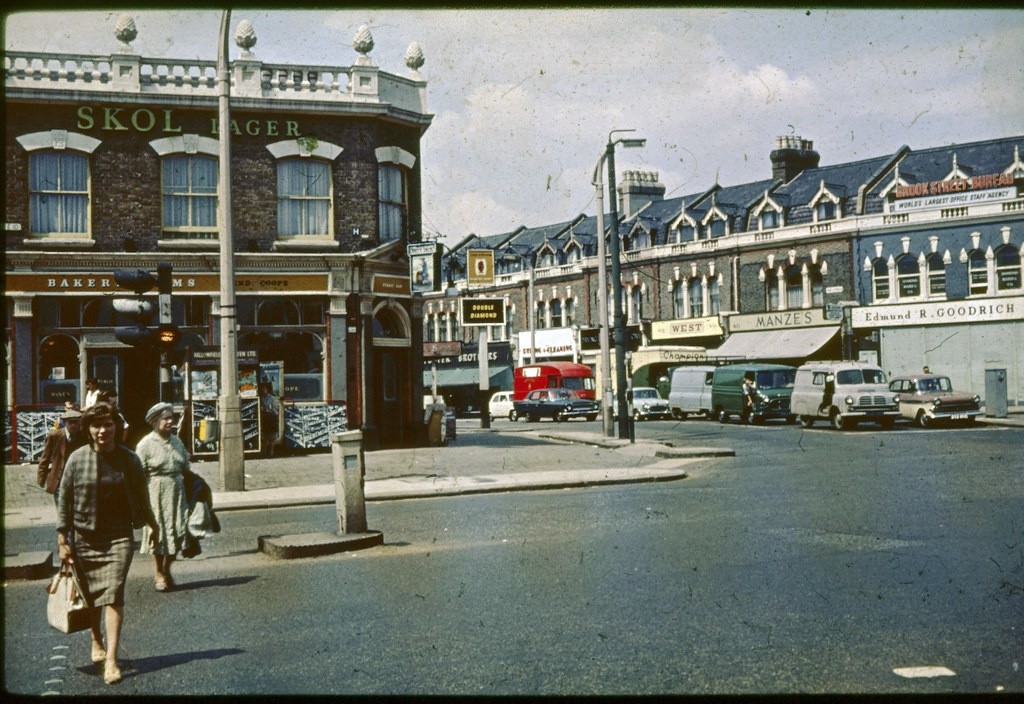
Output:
[592,138,648,435]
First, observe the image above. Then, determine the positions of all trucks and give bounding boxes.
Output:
[514,361,596,402]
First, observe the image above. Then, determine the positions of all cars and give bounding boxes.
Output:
[514,388,599,422]
[488,391,517,422]
[890,373,981,428]
[613,387,669,421]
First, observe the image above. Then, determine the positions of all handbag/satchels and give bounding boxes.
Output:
[44,553,92,635]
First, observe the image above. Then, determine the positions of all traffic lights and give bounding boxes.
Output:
[40,333,73,365]
[156,326,179,348]
[113,267,155,345]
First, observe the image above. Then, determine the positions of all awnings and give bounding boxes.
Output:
[715,326,841,360]
[423,366,510,387]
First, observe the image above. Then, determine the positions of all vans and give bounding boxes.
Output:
[669,365,716,420]
[789,359,900,430]
[712,363,796,425]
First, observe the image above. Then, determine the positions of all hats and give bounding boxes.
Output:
[60,409,83,421]
[144,402,174,422]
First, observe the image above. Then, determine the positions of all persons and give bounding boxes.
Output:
[55,400,75,429]
[85,377,101,408]
[36,410,84,546]
[924,366,938,389]
[96,389,124,440]
[419,257,428,285]
[55,402,159,685]
[740,379,754,424]
[134,402,190,591]
[259,383,278,458]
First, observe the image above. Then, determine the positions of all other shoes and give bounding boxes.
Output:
[103,660,121,685]
[91,641,106,663]
[154,576,176,591]
